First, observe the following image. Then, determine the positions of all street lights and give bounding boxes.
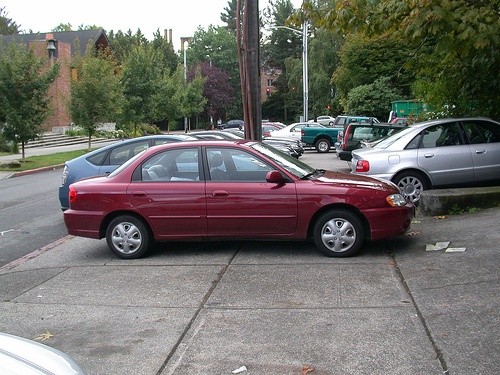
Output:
[183,41,189,133]
[206,54,213,124]
[265,21,309,122]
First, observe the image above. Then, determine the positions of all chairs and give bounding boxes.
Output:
[211,154,227,180]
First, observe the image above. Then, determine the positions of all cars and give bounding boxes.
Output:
[63,138,417,258]
[217,119,244,130]
[185,99,457,162]
[58,134,273,212]
[350,116,500,207]
